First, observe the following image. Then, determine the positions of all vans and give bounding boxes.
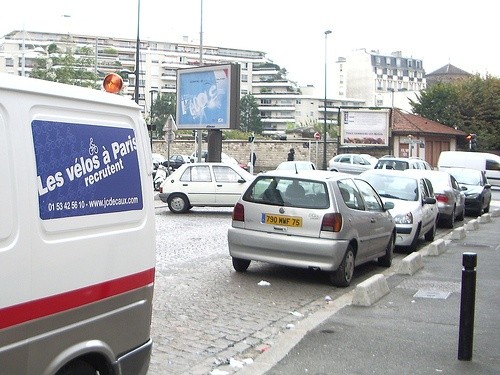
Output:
[1,73,157,374]
[436,151,500,187]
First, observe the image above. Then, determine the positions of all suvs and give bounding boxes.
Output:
[375,155,433,170]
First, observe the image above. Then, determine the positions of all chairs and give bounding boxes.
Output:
[262,184,357,208]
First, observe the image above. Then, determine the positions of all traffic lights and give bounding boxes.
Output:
[467,134,476,150]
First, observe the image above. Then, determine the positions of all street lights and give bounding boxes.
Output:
[323,30,333,169]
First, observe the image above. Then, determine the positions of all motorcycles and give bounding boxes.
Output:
[152,162,167,191]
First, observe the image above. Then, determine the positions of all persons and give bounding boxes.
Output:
[286,148,295,161]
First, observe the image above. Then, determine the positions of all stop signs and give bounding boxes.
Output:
[314,132,321,141]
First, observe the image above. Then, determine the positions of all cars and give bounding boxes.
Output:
[406,168,468,228]
[358,169,438,253]
[190,150,238,164]
[277,161,318,173]
[163,154,189,168]
[159,163,257,213]
[227,164,397,286]
[326,154,378,174]
[446,167,492,215]
